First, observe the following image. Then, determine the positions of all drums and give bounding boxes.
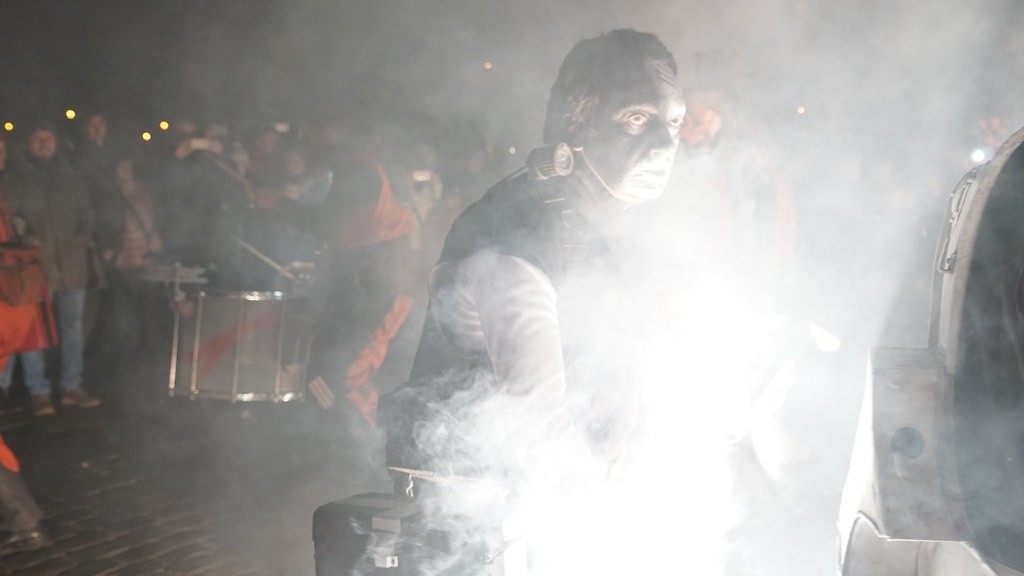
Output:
[171,288,306,405]
[936,121,1024,576]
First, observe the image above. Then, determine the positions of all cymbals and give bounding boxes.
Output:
[139,261,209,285]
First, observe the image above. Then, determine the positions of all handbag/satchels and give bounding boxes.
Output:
[0,264,47,306]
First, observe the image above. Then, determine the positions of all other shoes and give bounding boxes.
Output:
[33,405,56,415]
[59,392,101,408]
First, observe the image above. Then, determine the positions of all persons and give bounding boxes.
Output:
[379,30,686,576]
[0,111,726,556]
[839,124,1024,576]
